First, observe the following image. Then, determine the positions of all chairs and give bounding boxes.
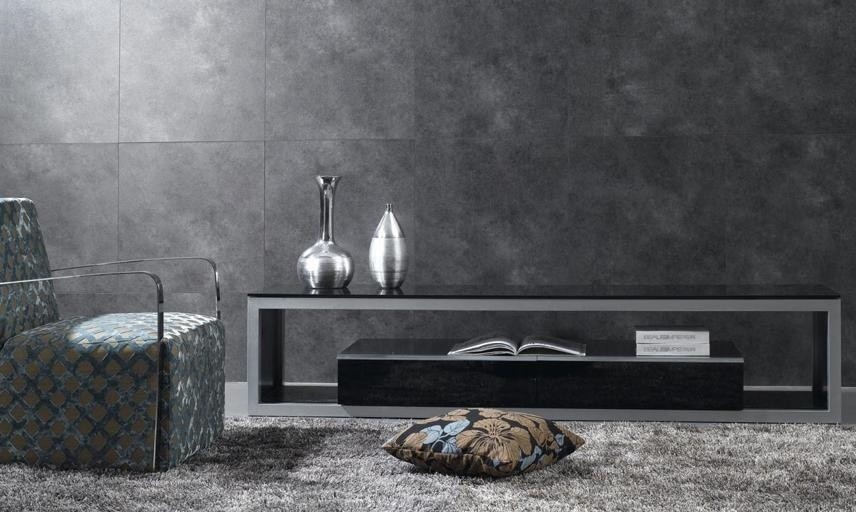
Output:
[1,197,225,472]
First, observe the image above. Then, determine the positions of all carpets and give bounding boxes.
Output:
[1,418,856,512]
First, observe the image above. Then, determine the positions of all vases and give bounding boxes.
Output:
[297,175,354,289]
[368,203,408,289]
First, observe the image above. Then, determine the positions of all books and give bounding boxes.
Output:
[447,330,586,357]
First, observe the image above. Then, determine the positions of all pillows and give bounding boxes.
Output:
[382,405,585,477]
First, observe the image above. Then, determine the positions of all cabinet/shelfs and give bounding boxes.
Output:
[244,288,842,426]
